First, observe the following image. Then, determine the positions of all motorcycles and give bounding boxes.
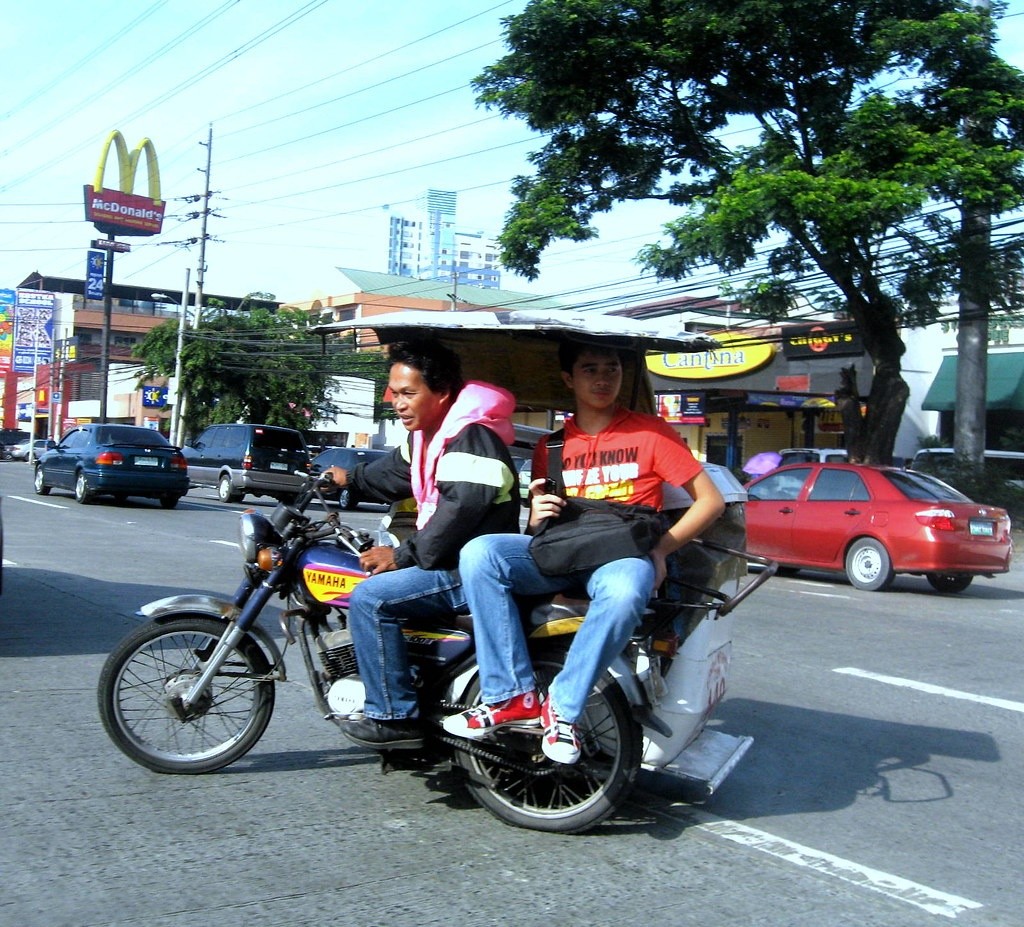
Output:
[94,464,776,835]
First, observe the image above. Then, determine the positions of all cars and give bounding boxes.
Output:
[310,447,403,509]
[9,439,59,463]
[31,425,191,509]
[736,464,1013,594]
[772,448,849,503]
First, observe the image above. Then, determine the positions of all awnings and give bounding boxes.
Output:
[919,353,1024,411]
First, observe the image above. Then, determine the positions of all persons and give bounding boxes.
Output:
[45,435,55,452]
[442,334,725,764]
[322,343,520,750]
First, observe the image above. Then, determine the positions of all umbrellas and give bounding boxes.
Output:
[743,451,782,476]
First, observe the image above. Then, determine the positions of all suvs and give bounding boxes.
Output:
[0,429,42,462]
[178,423,316,504]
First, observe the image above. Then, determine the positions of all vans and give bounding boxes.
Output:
[909,449,1023,500]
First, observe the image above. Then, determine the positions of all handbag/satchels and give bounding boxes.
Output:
[528,496,669,577]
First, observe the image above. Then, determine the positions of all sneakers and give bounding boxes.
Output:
[540,693,582,764]
[443,689,542,740]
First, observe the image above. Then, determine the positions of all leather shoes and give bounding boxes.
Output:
[340,718,424,749]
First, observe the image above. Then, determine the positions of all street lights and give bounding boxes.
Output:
[150,293,201,453]
